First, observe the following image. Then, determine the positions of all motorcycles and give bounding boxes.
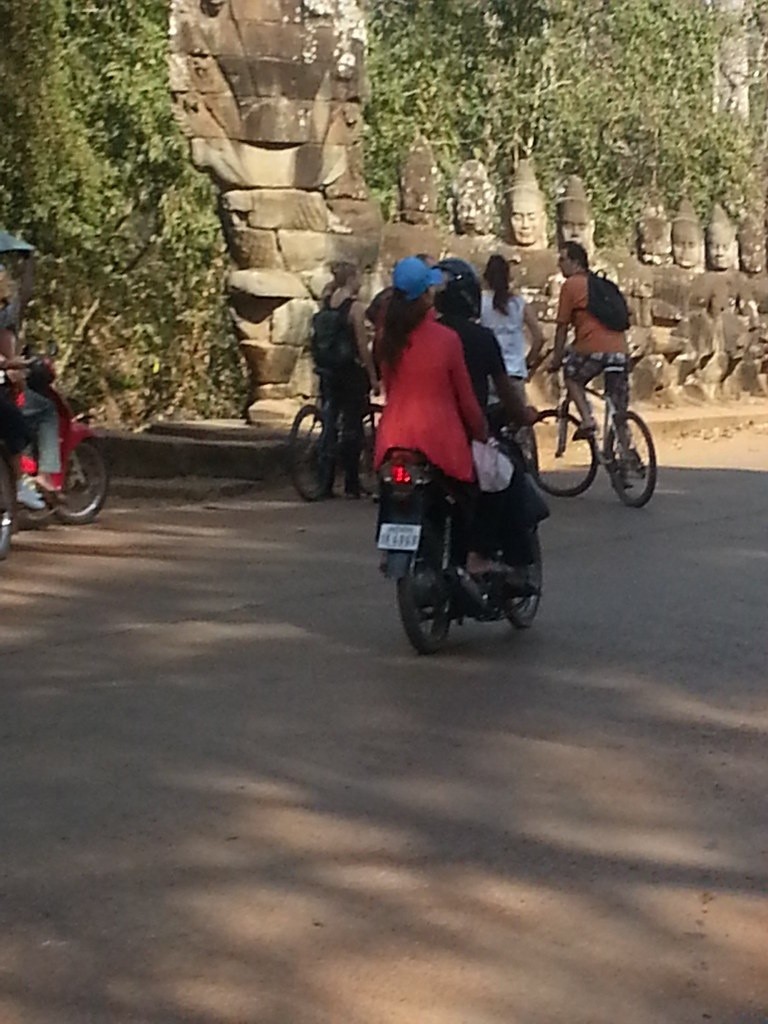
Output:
[374,399,549,656]
[0,344,110,561]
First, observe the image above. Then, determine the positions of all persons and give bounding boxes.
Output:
[310,254,546,576]
[0,271,62,508]
[635,196,768,275]
[393,153,598,252]
[548,241,641,464]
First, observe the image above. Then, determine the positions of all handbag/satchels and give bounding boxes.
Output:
[470,439,514,492]
[485,437,550,545]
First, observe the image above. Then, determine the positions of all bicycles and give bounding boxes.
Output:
[530,352,658,508]
[289,392,385,503]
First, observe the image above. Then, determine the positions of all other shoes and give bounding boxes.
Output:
[342,487,371,498]
[612,477,634,489]
[379,551,389,573]
[36,472,62,492]
[372,491,381,499]
[572,420,597,442]
[507,570,539,592]
[466,552,515,576]
[17,480,46,510]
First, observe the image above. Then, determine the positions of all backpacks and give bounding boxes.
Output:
[574,269,632,331]
[311,295,359,381]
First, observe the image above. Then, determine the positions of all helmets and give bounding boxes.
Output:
[431,257,483,318]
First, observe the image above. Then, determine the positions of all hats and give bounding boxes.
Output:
[392,257,443,302]
[483,255,514,283]
